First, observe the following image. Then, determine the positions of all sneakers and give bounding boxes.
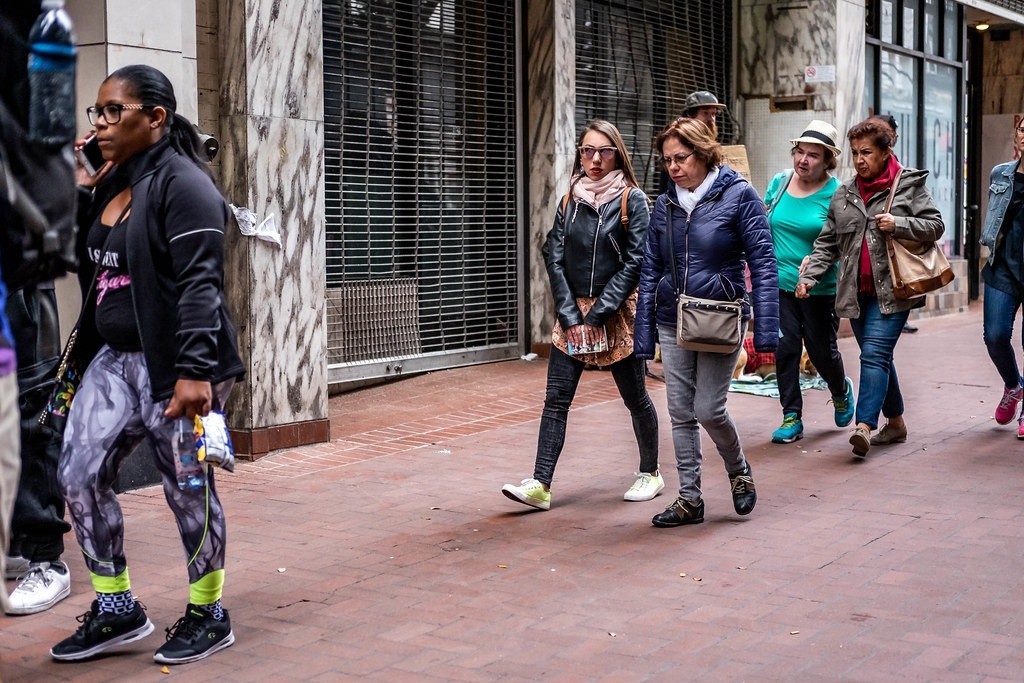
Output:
[6,560,71,614]
[995,375,1024,424]
[50,600,155,661]
[651,495,705,527]
[727,460,757,516]
[1016,410,1024,438]
[623,470,665,501]
[502,478,552,511]
[771,413,804,443]
[5,555,31,579]
[152,603,235,663]
[826,376,855,427]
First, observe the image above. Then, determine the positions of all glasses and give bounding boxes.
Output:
[659,150,696,169]
[87,104,150,125]
[578,146,618,159]
[1016,127,1024,135]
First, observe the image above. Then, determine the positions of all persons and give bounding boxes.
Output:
[734,337,817,379]
[50,65,249,664]
[978,116,1024,438]
[764,120,854,442]
[794,115,945,457]
[502,121,664,509]
[0,146,72,614]
[681,91,726,138]
[637,118,779,526]
[0,290,20,614]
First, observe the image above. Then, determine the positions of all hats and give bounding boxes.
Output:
[685,90,726,110]
[789,119,841,157]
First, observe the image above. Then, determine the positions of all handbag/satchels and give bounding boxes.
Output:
[38,328,83,434]
[883,168,955,298]
[676,291,742,354]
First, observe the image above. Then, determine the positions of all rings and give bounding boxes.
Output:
[588,329,592,330]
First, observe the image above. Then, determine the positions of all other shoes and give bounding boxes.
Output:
[848,424,871,457]
[870,418,907,445]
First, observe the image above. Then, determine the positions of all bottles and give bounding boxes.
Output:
[29,0,77,144]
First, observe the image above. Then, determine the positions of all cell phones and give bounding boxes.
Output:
[76,131,107,178]
